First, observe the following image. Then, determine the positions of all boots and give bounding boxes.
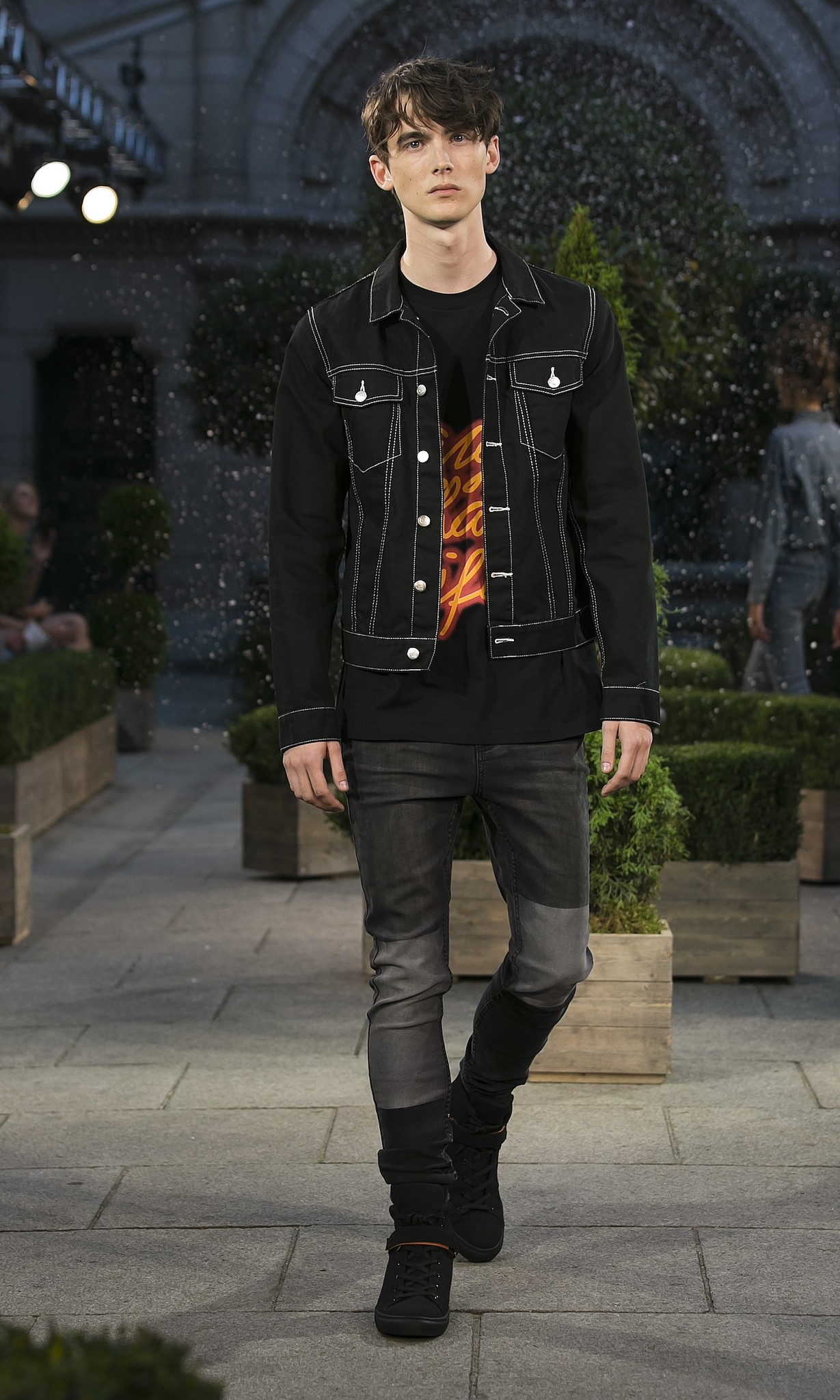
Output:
[374,1182,458,1339]
[445,1071,514,1263]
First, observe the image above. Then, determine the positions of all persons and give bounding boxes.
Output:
[0,475,90,651]
[265,56,661,1341]
[736,320,838,700]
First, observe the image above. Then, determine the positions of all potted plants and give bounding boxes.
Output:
[650,740,805,985]
[222,702,360,879]
[646,557,840,884]
[329,778,515,985]
[522,729,696,1087]
[2,481,174,949]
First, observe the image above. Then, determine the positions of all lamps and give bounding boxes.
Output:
[67,173,120,227]
[1,140,71,214]
